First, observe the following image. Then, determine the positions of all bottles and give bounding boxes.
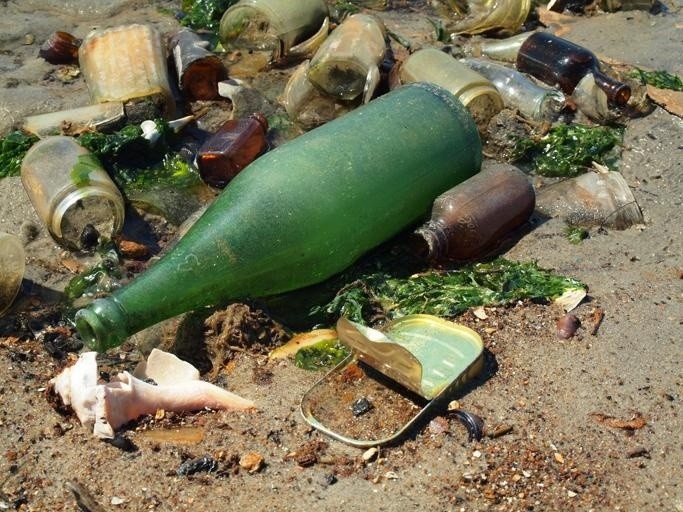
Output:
[193,111,270,190]
[514,32,631,107]
[411,162,535,266]
[73,83,484,349]
[465,55,563,129]
[309,11,380,100]
[163,29,231,96]
[532,169,641,228]
[80,25,173,117]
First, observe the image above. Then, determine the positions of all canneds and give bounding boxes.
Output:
[21,132,126,252]
[216,1,325,63]
[299,311,485,453]
[396,46,504,114]
